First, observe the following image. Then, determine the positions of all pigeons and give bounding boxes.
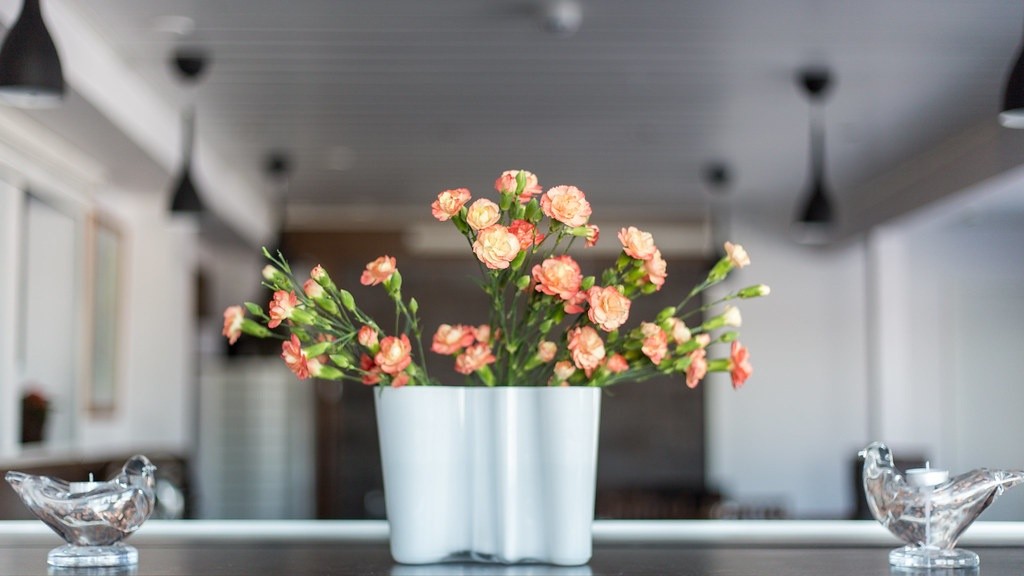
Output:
[5,451,159,545]
[856,438,1019,549]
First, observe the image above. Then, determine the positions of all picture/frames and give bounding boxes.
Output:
[89,212,123,417]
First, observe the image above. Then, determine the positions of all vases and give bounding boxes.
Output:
[373,385,600,567]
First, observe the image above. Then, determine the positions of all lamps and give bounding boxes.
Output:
[998,40,1024,130]
[169,48,211,215]
[1,0,65,104]
[791,66,835,221]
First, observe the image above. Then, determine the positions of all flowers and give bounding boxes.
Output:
[220,171,771,386]
